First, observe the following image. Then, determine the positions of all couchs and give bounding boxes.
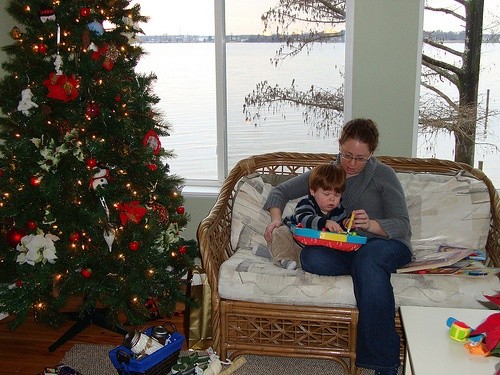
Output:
[197,152,500,375]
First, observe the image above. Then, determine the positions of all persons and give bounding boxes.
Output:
[266,163,355,272]
[263,118,413,375]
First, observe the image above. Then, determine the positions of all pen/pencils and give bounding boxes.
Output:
[347,210,356,234]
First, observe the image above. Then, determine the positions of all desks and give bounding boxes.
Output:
[398,306,500,375]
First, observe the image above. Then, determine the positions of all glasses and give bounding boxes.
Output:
[339,151,372,163]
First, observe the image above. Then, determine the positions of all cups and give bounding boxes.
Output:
[122,329,165,356]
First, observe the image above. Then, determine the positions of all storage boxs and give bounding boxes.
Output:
[109,321,185,375]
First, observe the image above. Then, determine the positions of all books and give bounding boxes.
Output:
[396,244,487,276]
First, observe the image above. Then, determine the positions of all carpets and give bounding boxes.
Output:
[56,343,403,375]
